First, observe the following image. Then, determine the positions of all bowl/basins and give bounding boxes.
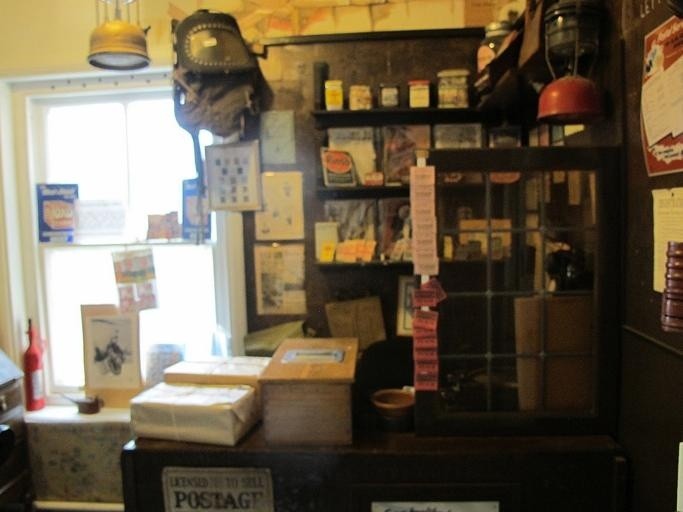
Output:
[371,389,416,431]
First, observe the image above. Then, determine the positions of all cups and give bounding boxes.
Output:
[76,398,104,415]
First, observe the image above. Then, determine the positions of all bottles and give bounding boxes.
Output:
[23,318,45,411]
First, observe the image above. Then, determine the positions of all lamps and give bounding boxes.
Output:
[89,0,150,70]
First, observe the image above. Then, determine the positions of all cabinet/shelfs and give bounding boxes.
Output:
[308,59,626,441]
[0,380,36,512]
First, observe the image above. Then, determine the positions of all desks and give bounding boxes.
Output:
[120,426,635,512]
